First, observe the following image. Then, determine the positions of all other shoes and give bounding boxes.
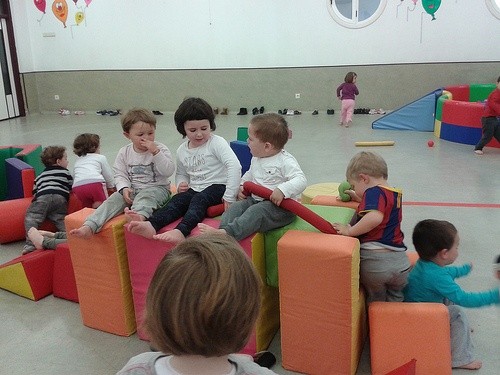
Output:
[214,107,218,115]
[252,107,258,114]
[278,109,288,115]
[260,105,264,114]
[96,109,122,117]
[237,107,248,115]
[152,111,163,115]
[221,108,228,115]
[312,110,318,115]
[327,109,334,115]
[354,109,369,114]
[294,110,301,115]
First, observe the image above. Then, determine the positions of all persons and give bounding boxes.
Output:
[337,72,359,128]
[115,232,277,375]
[473,76,500,155]
[27,226,68,251]
[67,132,117,214]
[403,219,500,370]
[128,98,242,246]
[197,113,307,243]
[68,107,176,240]
[22,145,74,255]
[331,151,410,303]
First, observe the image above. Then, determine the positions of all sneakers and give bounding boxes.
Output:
[370,108,386,115]
[287,110,294,115]
[253,350,277,368]
